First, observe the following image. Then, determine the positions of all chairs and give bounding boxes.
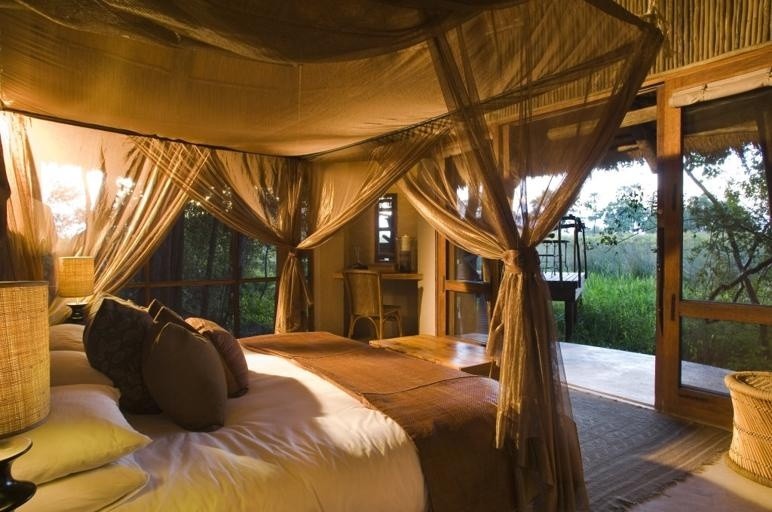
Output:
[343,270,405,344]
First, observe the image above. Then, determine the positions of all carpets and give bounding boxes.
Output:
[548,377,731,510]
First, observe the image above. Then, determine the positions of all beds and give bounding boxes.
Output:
[2,0,673,512]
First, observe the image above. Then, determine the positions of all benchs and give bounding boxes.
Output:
[367,332,493,374]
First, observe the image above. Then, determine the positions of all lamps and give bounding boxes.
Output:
[0,275,52,512]
[55,254,96,324]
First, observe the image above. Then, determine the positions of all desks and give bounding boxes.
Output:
[334,266,424,283]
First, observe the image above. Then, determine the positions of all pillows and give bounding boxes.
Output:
[10,451,149,512]
[45,323,89,350]
[186,315,250,399]
[146,294,188,334]
[5,380,153,486]
[82,290,162,416]
[143,306,227,434]
[44,346,117,388]
